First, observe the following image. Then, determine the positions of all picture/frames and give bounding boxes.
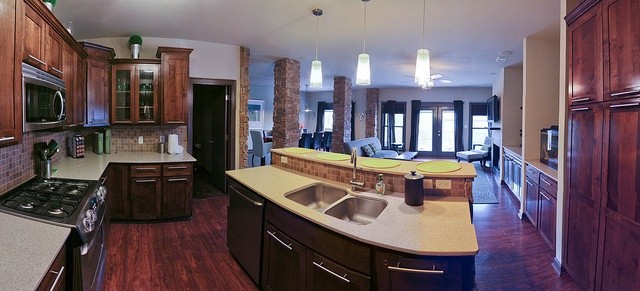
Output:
[248,99,265,130]
[156,45,194,127]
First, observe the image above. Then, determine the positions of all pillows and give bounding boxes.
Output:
[360,145,374,157]
[368,143,380,154]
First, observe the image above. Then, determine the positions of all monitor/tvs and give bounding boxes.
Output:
[487,95,498,124]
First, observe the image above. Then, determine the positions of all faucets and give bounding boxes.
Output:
[350,147,357,181]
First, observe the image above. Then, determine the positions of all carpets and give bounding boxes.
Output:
[472,163,499,204]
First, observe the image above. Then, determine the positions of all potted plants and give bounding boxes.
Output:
[129,34,142,58]
[42,0,57,13]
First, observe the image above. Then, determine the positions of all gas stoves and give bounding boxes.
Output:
[0,177,107,244]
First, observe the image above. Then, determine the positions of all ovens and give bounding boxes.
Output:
[21,62,65,133]
[79,205,106,291]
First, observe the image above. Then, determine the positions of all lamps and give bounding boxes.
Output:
[414,0,430,84]
[356,0,371,86]
[302,84,312,113]
[310,9,323,89]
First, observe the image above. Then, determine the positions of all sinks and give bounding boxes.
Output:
[323,197,389,226]
[283,182,348,214]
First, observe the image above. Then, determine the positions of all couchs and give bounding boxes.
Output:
[343,137,398,158]
[456,136,492,172]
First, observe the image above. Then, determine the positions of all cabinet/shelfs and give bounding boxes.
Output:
[0,0,23,145]
[563,0,640,290]
[61,41,88,129]
[524,164,557,254]
[131,164,193,221]
[375,251,474,291]
[262,221,371,290]
[108,58,161,126]
[227,178,265,288]
[79,41,116,129]
[503,151,522,205]
[23,0,61,78]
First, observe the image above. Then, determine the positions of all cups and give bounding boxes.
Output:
[159,143,165,153]
[40,160,50,180]
[404,170,424,206]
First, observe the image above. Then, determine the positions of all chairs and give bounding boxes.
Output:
[313,131,322,150]
[249,129,273,166]
[323,132,332,151]
[299,133,312,148]
